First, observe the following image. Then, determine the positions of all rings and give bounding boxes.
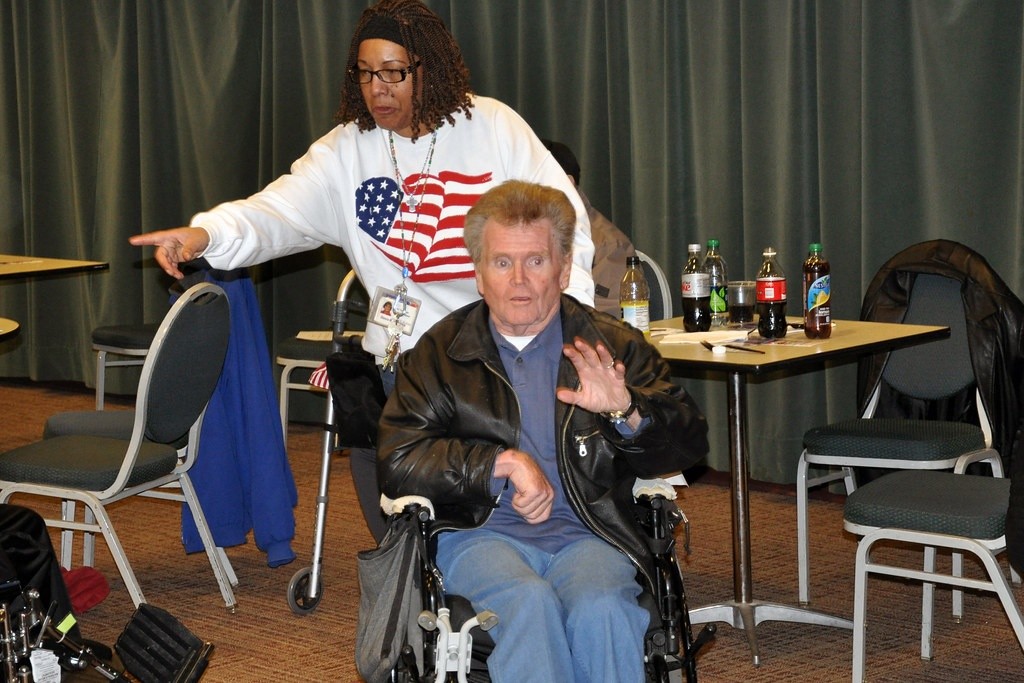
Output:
[605,361,615,369]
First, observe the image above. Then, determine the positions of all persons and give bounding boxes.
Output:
[381,303,391,316]
[378,181,710,683]
[537,136,644,323]
[129,1,596,541]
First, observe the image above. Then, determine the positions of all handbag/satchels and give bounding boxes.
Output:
[325,333,394,451]
[353,505,426,683]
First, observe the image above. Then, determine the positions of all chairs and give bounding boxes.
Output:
[0,243,1024,683]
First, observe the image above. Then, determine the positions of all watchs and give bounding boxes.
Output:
[597,384,636,423]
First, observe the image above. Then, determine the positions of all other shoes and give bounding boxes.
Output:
[36,638,113,671]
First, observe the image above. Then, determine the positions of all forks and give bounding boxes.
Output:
[701,341,766,354]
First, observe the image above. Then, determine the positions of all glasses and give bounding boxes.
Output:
[348,59,421,84]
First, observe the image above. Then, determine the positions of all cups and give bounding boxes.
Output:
[727,281,756,326]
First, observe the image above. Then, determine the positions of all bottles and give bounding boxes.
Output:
[801,243,831,339]
[682,243,712,333]
[618,255,651,343]
[757,247,787,339]
[700,239,729,328]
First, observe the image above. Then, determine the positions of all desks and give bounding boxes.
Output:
[614,306,947,664]
[0,317,21,356]
[0,251,112,303]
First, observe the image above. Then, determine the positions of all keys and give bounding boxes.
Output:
[381,335,402,372]
